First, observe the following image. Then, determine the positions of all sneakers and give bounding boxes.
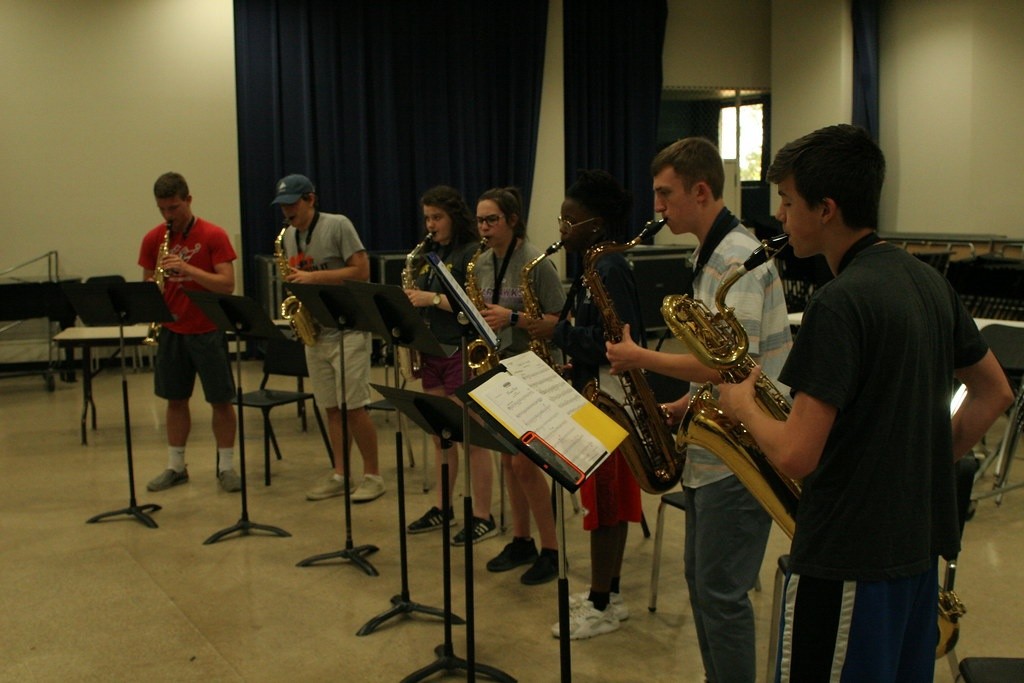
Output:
[350,475,385,500]
[551,600,621,638]
[568,590,628,620]
[450,514,497,546]
[407,506,455,532]
[305,477,357,499]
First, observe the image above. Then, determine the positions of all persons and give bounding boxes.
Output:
[528,166,643,638]
[138,171,243,494]
[406,185,500,544]
[465,188,570,586]
[603,136,794,683]
[267,175,387,503]
[712,121,1013,683]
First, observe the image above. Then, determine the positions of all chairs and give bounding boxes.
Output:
[216,325,335,487]
[365,398,430,494]
[647,491,762,614]
[79,275,143,374]
[954,656,1024,683]
[766,456,965,683]
[981,324,1024,491]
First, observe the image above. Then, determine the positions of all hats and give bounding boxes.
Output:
[271,174,316,204]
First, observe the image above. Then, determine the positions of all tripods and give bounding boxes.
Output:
[63,280,177,529]
[183,285,293,546]
[292,276,522,683]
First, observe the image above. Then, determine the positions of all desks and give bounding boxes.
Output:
[51,320,309,446]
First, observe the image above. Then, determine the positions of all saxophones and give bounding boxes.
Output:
[463,241,565,380]
[274,217,322,347]
[660,232,805,541]
[142,219,174,346]
[389,231,436,381]
[583,220,687,495]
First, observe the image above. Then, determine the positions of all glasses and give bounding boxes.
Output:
[558,215,597,234]
[473,214,507,228]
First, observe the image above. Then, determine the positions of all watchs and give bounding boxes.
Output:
[510,309,519,326]
[432,292,442,307]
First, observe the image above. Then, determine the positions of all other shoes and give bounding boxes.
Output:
[485,539,538,571]
[520,549,560,584]
[148,465,188,490]
[218,467,242,493]
